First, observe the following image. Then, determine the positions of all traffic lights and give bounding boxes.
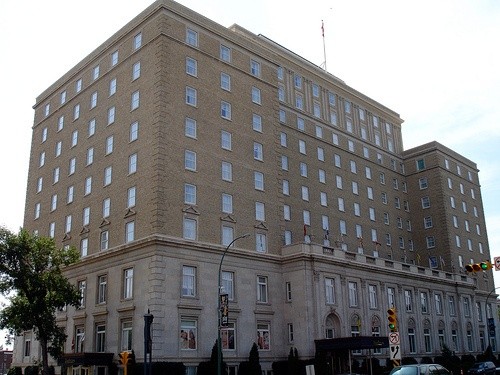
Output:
[118,351,126,368]
[387,308,397,333]
[465,261,492,273]
[126,352,133,366]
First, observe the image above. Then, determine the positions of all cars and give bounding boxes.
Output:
[471,361,500,375]
[388,364,455,375]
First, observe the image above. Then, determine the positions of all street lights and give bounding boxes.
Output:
[485,287,500,347]
[217,233,252,375]
